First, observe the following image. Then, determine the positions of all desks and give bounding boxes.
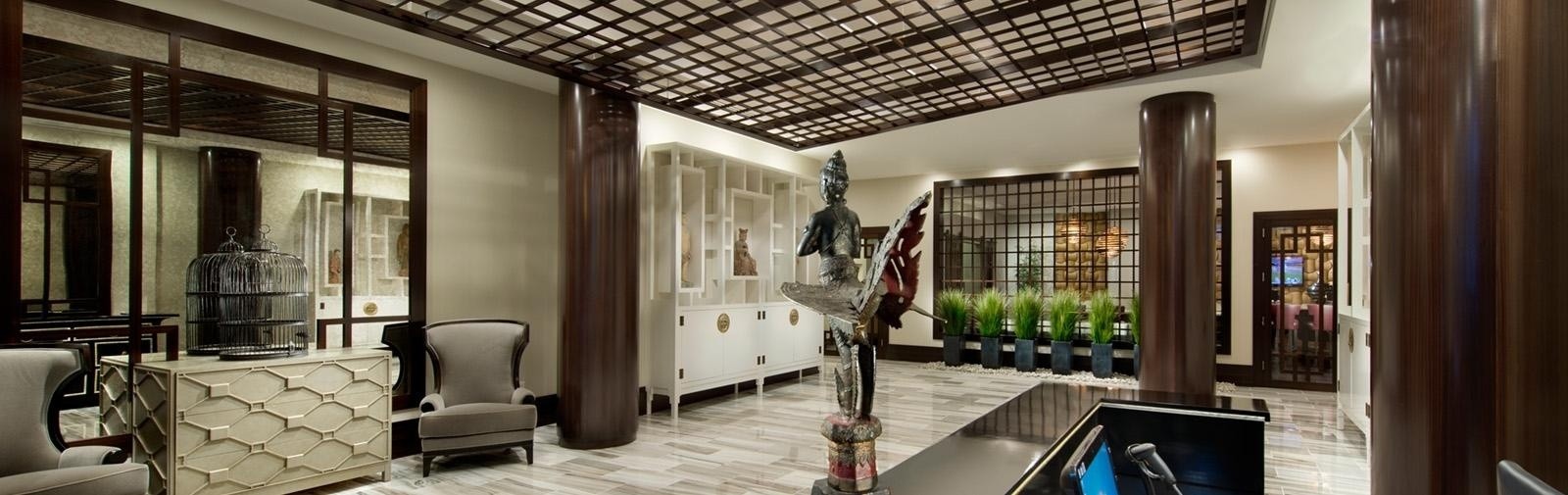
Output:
[1263,301,1334,354]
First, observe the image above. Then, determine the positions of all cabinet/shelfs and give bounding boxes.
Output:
[300,186,410,352]
[100,350,188,441]
[639,140,824,418]
[1333,100,1371,469]
[131,347,394,495]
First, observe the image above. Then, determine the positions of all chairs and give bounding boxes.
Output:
[381,322,412,399]
[417,316,538,477]
[0,342,150,495]
[1497,459,1565,495]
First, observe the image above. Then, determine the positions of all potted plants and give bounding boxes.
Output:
[1007,285,1048,371]
[968,285,1010,369]
[1125,290,1140,381]
[1044,286,1085,375]
[934,286,975,366]
[1087,288,1116,378]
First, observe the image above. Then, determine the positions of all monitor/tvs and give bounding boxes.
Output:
[1271,254,1305,286]
[1060,424,1122,495]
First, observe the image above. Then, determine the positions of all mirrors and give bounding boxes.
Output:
[0,0,427,469]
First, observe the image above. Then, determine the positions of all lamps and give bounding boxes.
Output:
[1059,180,1087,244]
[1096,177,1128,258]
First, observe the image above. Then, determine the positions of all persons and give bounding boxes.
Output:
[682,210,692,284]
[797,148,863,286]
[330,248,342,284]
[396,223,409,276]
[735,227,758,276]
